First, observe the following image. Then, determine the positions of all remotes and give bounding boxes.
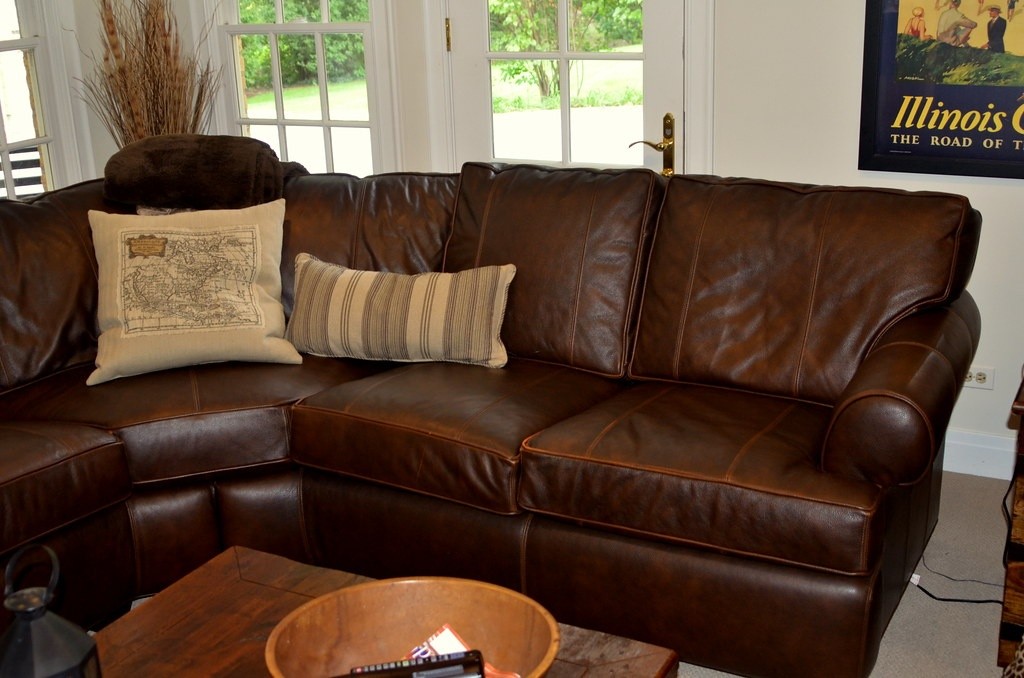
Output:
[351,650,484,678]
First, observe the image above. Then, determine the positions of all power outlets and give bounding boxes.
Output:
[962,367,995,390]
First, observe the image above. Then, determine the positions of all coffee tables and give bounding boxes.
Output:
[90,543,678,678]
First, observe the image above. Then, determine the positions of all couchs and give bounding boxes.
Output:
[0,133,982,678]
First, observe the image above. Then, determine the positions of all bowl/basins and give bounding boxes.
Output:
[264,575,562,678]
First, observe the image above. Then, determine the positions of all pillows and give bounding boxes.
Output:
[86,196,302,384]
[284,249,517,368]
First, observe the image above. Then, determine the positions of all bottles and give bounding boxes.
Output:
[0,543,104,678]
